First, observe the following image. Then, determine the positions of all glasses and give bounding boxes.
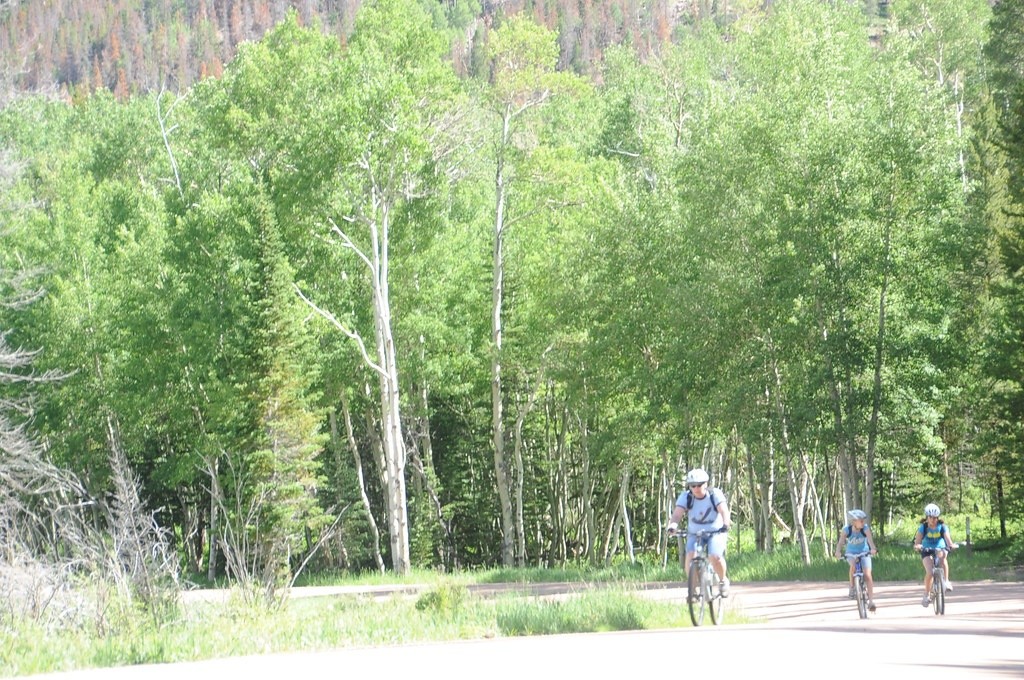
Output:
[691,483,704,488]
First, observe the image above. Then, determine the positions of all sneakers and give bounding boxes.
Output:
[922,595,929,607]
[850,588,853,596]
[687,594,697,602]
[720,580,729,597]
[869,602,875,608]
[945,584,952,590]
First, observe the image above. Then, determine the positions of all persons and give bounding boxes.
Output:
[834,510,876,611]
[915,503,955,607]
[665,468,733,602]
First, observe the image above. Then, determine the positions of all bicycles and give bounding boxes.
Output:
[668,526,729,627]
[833,550,878,620]
[920,546,958,616]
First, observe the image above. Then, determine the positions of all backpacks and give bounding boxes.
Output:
[913,531,917,543]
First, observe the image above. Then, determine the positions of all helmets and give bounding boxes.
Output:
[848,509,866,518]
[924,503,940,518]
[687,470,709,482]
